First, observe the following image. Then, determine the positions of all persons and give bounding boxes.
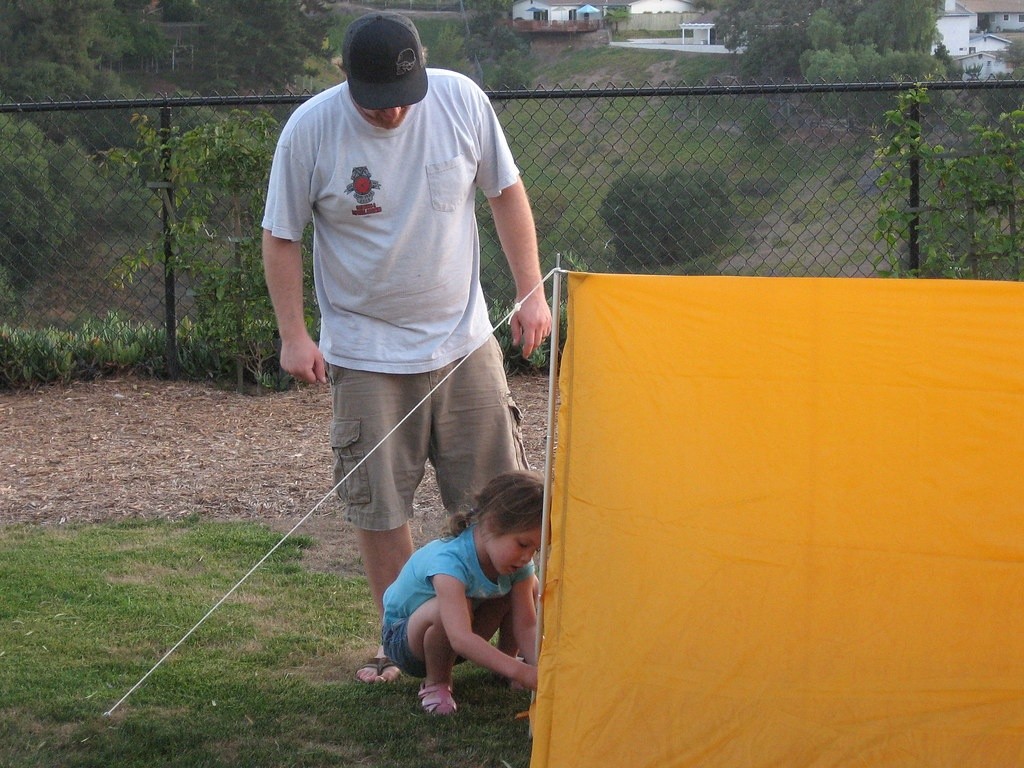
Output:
[382,471,554,712]
[262,9,553,688]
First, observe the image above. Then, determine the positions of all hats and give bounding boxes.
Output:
[344,11,429,111]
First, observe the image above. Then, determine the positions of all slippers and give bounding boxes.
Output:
[353,654,404,686]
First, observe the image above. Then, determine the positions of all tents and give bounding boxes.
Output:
[104,267,1024,768]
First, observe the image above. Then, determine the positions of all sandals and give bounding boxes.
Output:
[417,681,457,717]
[495,671,525,692]
